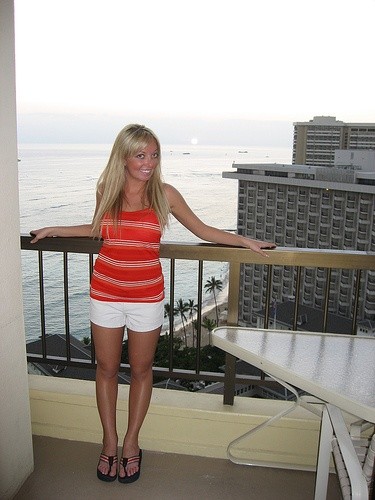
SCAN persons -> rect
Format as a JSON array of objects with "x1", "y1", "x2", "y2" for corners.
[{"x1": 30, "y1": 124, "x2": 276, "y2": 483}]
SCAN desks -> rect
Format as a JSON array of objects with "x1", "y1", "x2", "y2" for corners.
[{"x1": 211, "y1": 326, "x2": 375, "y2": 500}]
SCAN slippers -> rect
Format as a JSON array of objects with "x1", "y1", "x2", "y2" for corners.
[
  {"x1": 96, "y1": 454, "x2": 118, "y2": 482},
  {"x1": 118, "y1": 449, "x2": 142, "y2": 484}
]
